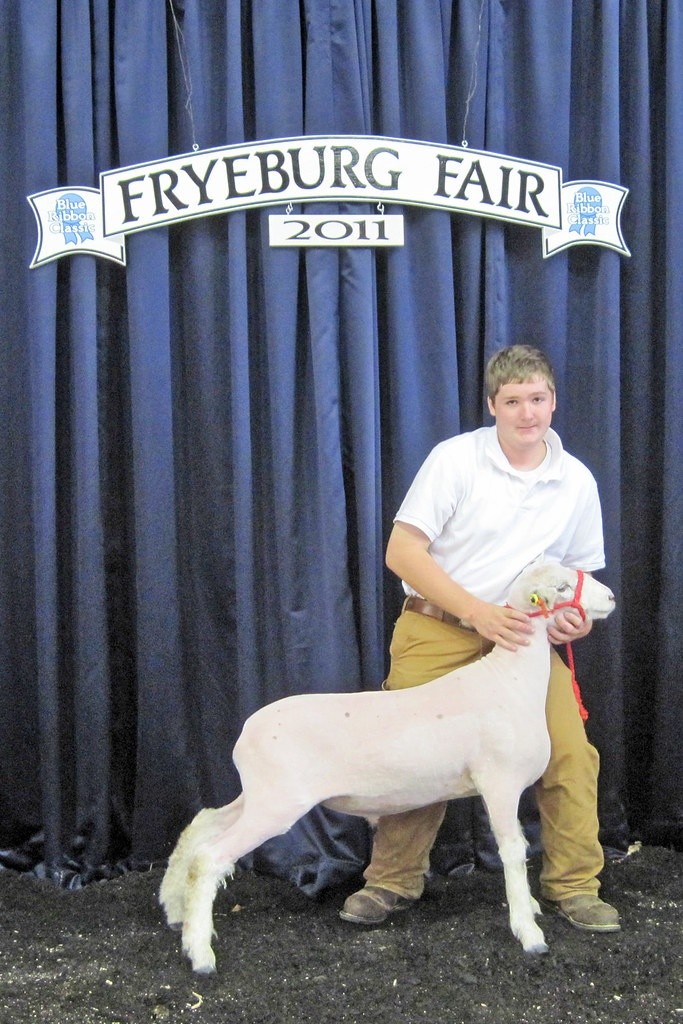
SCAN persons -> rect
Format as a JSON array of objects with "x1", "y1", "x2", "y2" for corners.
[{"x1": 338, "y1": 344, "x2": 624, "y2": 933}]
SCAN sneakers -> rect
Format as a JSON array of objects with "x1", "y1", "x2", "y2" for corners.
[
  {"x1": 539, "y1": 894, "x2": 621, "y2": 933},
  {"x1": 338, "y1": 886, "x2": 414, "y2": 924}
]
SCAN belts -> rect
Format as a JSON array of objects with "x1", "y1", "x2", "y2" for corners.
[{"x1": 405, "y1": 597, "x2": 478, "y2": 633}]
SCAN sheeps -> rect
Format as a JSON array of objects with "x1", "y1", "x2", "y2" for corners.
[{"x1": 160, "y1": 560, "x2": 617, "y2": 978}]
